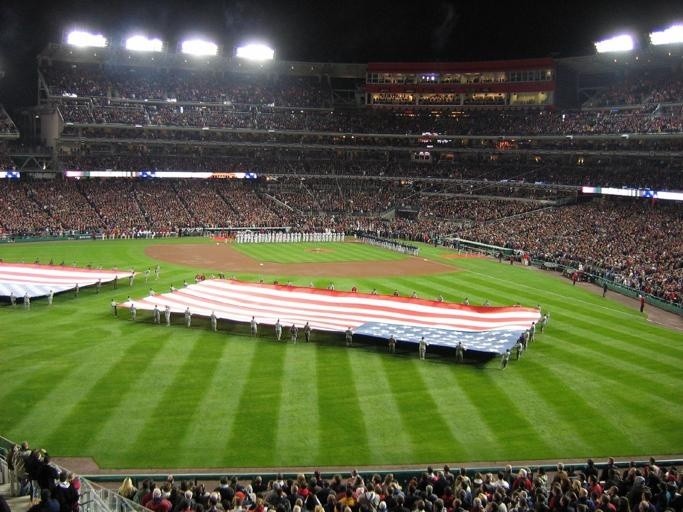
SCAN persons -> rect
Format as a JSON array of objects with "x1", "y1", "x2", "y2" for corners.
[
  {"x1": 0, "y1": 439, "x2": 80, "y2": 510},
  {"x1": 0, "y1": 256, "x2": 161, "y2": 311}
]
